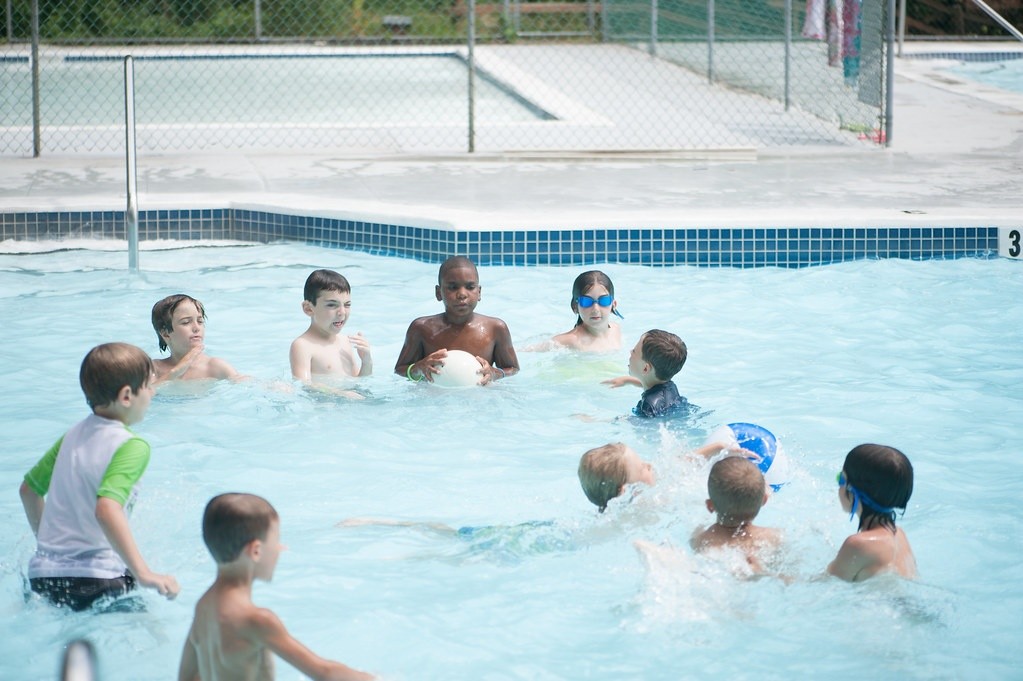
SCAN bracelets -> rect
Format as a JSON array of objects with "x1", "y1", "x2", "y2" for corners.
[
  {"x1": 406, "y1": 365, "x2": 421, "y2": 382},
  {"x1": 497, "y1": 368, "x2": 506, "y2": 378}
]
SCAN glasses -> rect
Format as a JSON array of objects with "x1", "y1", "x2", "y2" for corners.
[
  {"x1": 837, "y1": 470, "x2": 848, "y2": 487},
  {"x1": 575, "y1": 295, "x2": 612, "y2": 308}
]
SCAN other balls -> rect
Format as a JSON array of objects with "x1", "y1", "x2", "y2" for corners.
[{"x1": 427, "y1": 349, "x2": 484, "y2": 390}]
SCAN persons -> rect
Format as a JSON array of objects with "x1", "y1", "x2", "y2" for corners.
[
  {"x1": 176, "y1": 493, "x2": 379, "y2": 681},
  {"x1": 393, "y1": 256, "x2": 519, "y2": 385},
  {"x1": 556, "y1": 270, "x2": 625, "y2": 351},
  {"x1": 576, "y1": 330, "x2": 800, "y2": 574},
  {"x1": 291, "y1": 268, "x2": 374, "y2": 380},
  {"x1": 19, "y1": 343, "x2": 181, "y2": 617},
  {"x1": 826, "y1": 445, "x2": 917, "y2": 583},
  {"x1": 142, "y1": 293, "x2": 250, "y2": 380}
]
